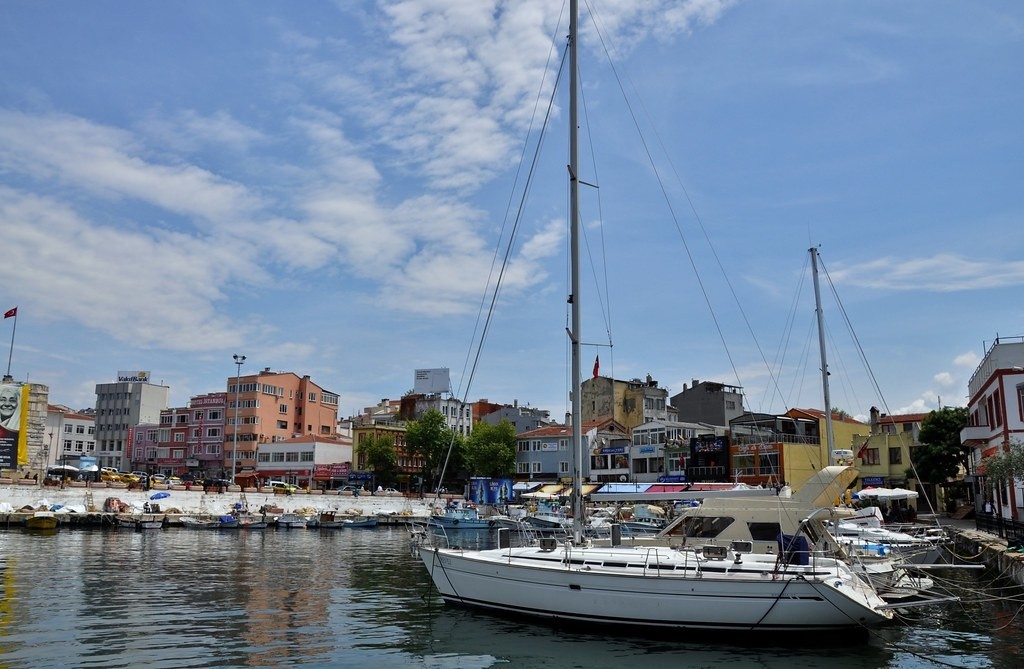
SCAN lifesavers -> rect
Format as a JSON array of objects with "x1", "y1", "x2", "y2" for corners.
[
  {"x1": 489, "y1": 520, "x2": 496, "y2": 527},
  {"x1": 453, "y1": 519, "x2": 460, "y2": 524}
]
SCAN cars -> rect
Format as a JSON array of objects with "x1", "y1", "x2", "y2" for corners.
[
  {"x1": 336, "y1": 486, "x2": 361, "y2": 497},
  {"x1": 376, "y1": 488, "x2": 398, "y2": 496},
  {"x1": 100, "y1": 466, "x2": 304, "y2": 495},
  {"x1": 47, "y1": 467, "x2": 84, "y2": 481}
]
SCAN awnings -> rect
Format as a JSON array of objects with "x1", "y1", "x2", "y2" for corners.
[
  {"x1": 973, "y1": 459, "x2": 989, "y2": 478},
  {"x1": 234, "y1": 473, "x2": 260, "y2": 478},
  {"x1": 511, "y1": 482, "x2": 738, "y2": 497}
]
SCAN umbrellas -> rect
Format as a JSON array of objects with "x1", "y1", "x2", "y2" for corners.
[
  {"x1": 840, "y1": 485, "x2": 919, "y2": 511},
  {"x1": 521, "y1": 491, "x2": 559, "y2": 505},
  {"x1": 41, "y1": 464, "x2": 107, "y2": 474}
]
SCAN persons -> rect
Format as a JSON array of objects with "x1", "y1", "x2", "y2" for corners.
[
  {"x1": 0, "y1": 384, "x2": 22, "y2": 429},
  {"x1": 434, "y1": 485, "x2": 450, "y2": 494},
  {"x1": 245, "y1": 477, "x2": 262, "y2": 493},
  {"x1": 881, "y1": 504, "x2": 916, "y2": 523},
  {"x1": 138, "y1": 475, "x2": 194, "y2": 491},
  {"x1": 0, "y1": 467, "x2": 90, "y2": 487},
  {"x1": 982, "y1": 497, "x2": 997, "y2": 534},
  {"x1": 360, "y1": 483, "x2": 386, "y2": 493}
]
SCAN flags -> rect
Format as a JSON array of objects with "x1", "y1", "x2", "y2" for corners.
[{"x1": 592, "y1": 354, "x2": 600, "y2": 379}]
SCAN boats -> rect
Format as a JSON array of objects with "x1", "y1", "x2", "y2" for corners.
[
  {"x1": 115, "y1": 516, "x2": 162, "y2": 530},
  {"x1": 23, "y1": 517, "x2": 58, "y2": 530},
  {"x1": 178, "y1": 513, "x2": 377, "y2": 529}
]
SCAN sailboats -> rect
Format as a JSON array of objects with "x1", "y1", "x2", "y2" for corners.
[{"x1": 409, "y1": 0, "x2": 963, "y2": 631}]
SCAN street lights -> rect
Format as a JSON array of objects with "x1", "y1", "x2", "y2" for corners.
[{"x1": 233, "y1": 354, "x2": 247, "y2": 485}]
